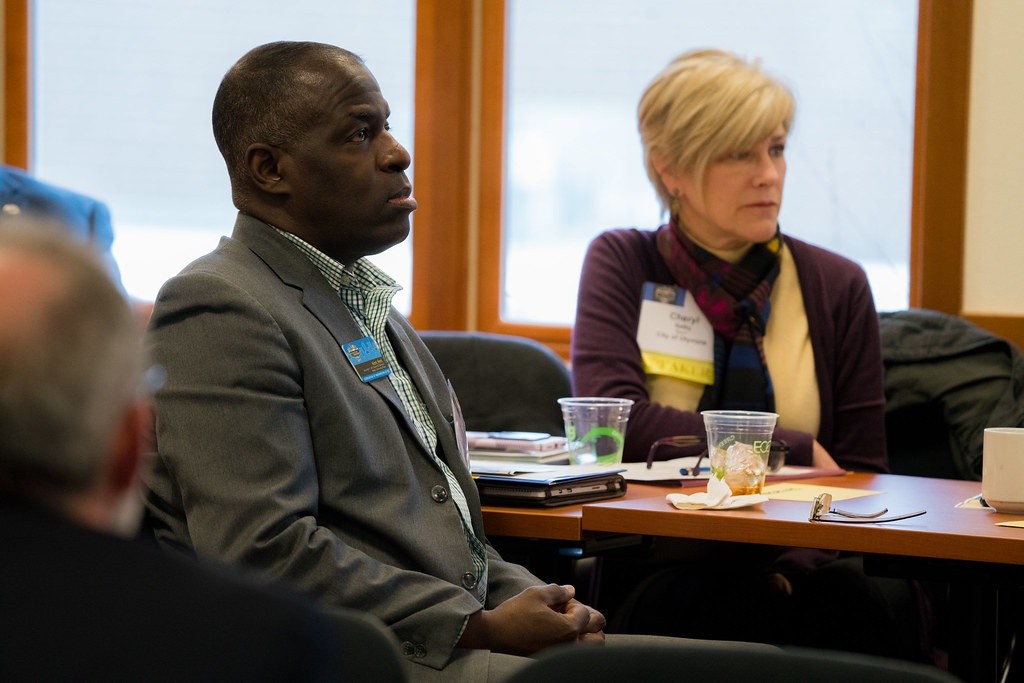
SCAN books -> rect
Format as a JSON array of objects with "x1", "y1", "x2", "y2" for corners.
[{"x1": 467, "y1": 430, "x2": 628, "y2": 510}]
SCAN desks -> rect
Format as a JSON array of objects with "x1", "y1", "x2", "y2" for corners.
[{"x1": 467, "y1": 460, "x2": 1024, "y2": 683}]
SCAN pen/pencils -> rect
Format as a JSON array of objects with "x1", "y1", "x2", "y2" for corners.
[{"x1": 679, "y1": 467, "x2": 711, "y2": 476}]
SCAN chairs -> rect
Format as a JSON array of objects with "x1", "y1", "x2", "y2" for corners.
[{"x1": 413, "y1": 330, "x2": 574, "y2": 441}]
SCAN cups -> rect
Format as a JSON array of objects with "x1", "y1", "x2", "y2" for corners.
[
  {"x1": 701, "y1": 409, "x2": 780, "y2": 496},
  {"x1": 559, "y1": 396, "x2": 634, "y2": 467},
  {"x1": 981, "y1": 427, "x2": 1024, "y2": 514}
]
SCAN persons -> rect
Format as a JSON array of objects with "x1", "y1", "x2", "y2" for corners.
[
  {"x1": 132, "y1": 44, "x2": 782, "y2": 683},
  {"x1": 0, "y1": 214, "x2": 409, "y2": 683},
  {"x1": 571, "y1": 48, "x2": 952, "y2": 672}
]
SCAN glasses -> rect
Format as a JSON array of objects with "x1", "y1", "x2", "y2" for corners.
[
  {"x1": 645, "y1": 436, "x2": 791, "y2": 476},
  {"x1": 807, "y1": 491, "x2": 928, "y2": 527}
]
